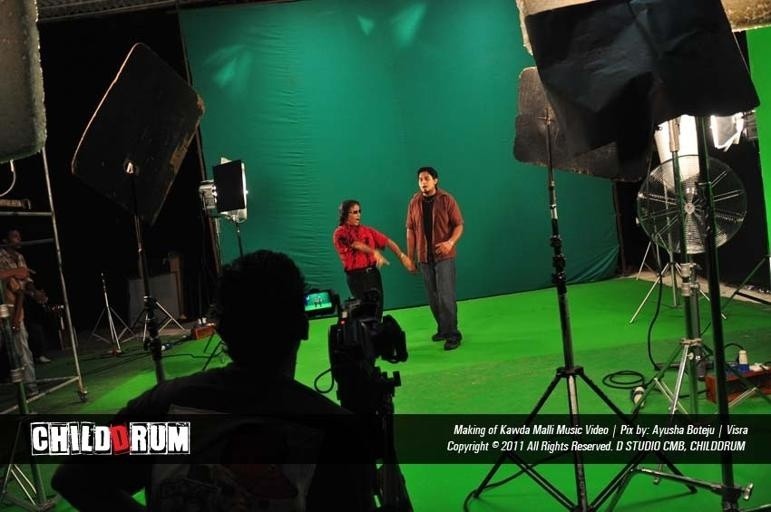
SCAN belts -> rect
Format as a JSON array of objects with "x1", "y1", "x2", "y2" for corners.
[{"x1": 347, "y1": 266, "x2": 376, "y2": 275}]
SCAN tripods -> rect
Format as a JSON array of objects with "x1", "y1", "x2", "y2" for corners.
[
  {"x1": 627, "y1": 261, "x2": 771, "y2": 483},
  {"x1": 630, "y1": 188, "x2": 727, "y2": 324},
  {"x1": 90, "y1": 271, "x2": 135, "y2": 354},
  {"x1": 127, "y1": 228, "x2": 187, "y2": 347},
  {"x1": 475, "y1": 237, "x2": 695, "y2": 512}
]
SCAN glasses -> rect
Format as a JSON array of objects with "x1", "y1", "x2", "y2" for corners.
[{"x1": 344, "y1": 209, "x2": 362, "y2": 215}]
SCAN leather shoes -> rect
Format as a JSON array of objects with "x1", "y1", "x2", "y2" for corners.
[
  {"x1": 432, "y1": 333, "x2": 446, "y2": 341},
  {"x1": 444, "y1": 340, "x2": 458, "y2": 350}
]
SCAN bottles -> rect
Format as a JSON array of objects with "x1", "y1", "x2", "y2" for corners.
[
  {"x1": 633, "y1": 387, "x2": 647, "y2": 408},
  {"x1": 738, "y1": 350, "x2": 749, "y2": 371},
  {"x1": 161, "y1": 343, "x2": 172, "y2": 352}
]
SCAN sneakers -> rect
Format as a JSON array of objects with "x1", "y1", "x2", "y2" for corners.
[{"x1": 34, "y1": 355, "x2": 52, "y2": 363}]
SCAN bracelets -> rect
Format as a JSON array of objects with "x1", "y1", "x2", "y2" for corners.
[
  {"x1": 397, "y1": 253, "x2": 405, "y2": 261},
  {"x1": 447, "y1": 240, "x2": 456, "y2": 247}
]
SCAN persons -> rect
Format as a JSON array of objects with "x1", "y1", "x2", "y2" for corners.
[
  {"x1": 1, "y1": 277, "x2": 39, "y2": 397},
  {"x1": 51, "y1": 248, "x2": 376, "y2": 512},
  {"x1": 407, "y1": 167, "x2": 464, "y2": 350},
  {"x1": 0, "y1": 229, "x2": 51, "y2": 363},
  {"x1": 333, "y1": 199, "x2": 417, "y2": 318}
]
